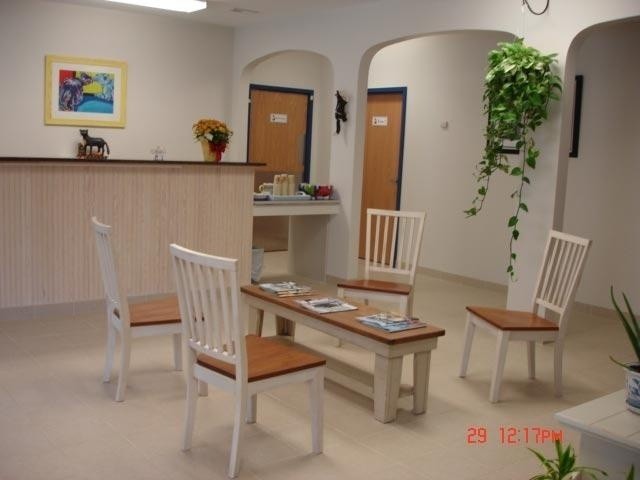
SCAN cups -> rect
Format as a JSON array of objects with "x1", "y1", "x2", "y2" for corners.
[{"x1": 258, "y1": 174, "x2": 332, "y2": 200}]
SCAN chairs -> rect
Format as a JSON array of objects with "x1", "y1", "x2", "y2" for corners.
[
  {"x1": 335, "y1": 205, "x2": 425, "y2": 351},
  {"x1": 168, "y1": 241, "x2": 326, "y2": 479},
  {"x1": 460, "y1": 226, "x2": 593, "y2": 406},
  {"x1": 89, "y1": 214, "x2": 210, "y2": 403}
]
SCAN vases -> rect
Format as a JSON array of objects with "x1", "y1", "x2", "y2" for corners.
[{"x1": 200, "y1": 141, "x2": 222, "y2": 163}]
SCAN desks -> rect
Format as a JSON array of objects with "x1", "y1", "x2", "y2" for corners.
[{"x1": 240, "y1": 282, "x2": 445, "y2": 425}]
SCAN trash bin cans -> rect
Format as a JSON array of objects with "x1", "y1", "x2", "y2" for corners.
[{"x1": 252, "y1": 247, "x2": 266, "y2": 281}]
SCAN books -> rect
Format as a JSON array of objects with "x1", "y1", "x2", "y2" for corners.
[
  {"x1": 260, "y1": 281, "x2": 319, "y2": 298},
  {"x1": 356, "y1": 310, "x2": 428, "y2": 333},
  {"x1": 293, "y1": 298, "x2": 358, "y2": 314}
]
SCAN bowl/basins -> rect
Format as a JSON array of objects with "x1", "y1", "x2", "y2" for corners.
[{"x1": 253, "y1": 194, "x2": 268, "y2": 200}]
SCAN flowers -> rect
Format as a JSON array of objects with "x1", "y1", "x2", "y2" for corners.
[{"x1": 191, "y1": 118, "x2": 234, "y2": 162}]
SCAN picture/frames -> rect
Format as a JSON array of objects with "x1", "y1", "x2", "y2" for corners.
[{"x1": 42, "y1": 53, "x2": 131, "y2": 129}]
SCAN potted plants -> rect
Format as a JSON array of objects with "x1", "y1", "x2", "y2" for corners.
[{"x1": 603, "y1": 284, "x2": 639, "y2": 416}]
